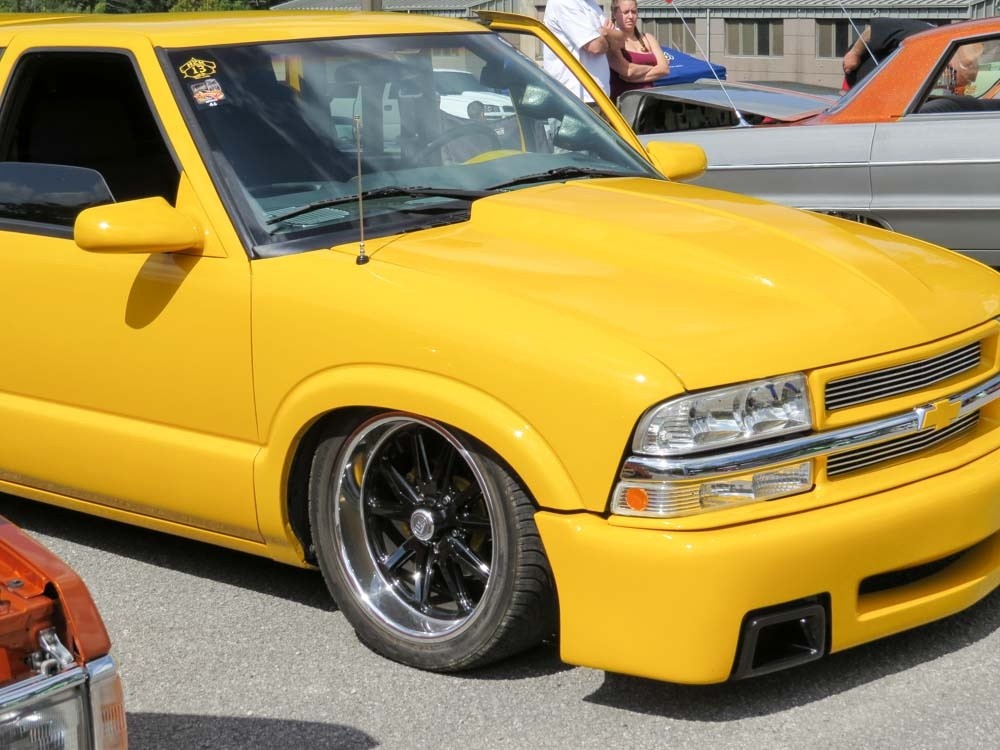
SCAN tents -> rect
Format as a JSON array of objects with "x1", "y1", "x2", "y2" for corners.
[{"x1": 652, "y1": 46, "x2": 726, "y2": 87}]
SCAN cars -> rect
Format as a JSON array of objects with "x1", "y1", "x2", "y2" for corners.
[
  {"x1": 0, "y1": 504, "x2": 129, "y2": 750},
  {"x1": 2, "y1": 11, "x2": 1000, "y2": 686}
]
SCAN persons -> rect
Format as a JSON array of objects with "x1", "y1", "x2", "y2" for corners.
[
  {"x1": 608, "y1": 0, "x2": 670, "y2": 106},
  {"x1": 842, "y1": 16, "x2": 985, "y2": 95},
  {"x1": 543, "y1": 0, "x2": 626, "y2": 157},
  {"x1": 467, "y1": 101, "x2": 486, "y2": 121}
]
